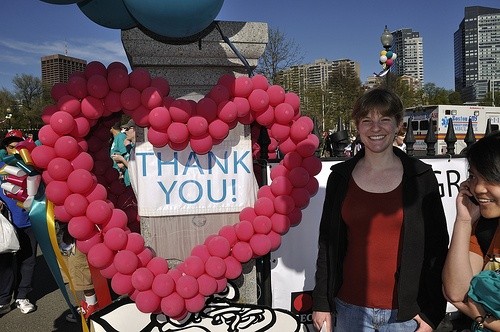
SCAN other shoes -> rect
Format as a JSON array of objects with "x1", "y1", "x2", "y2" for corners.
[{"x1": 66, "y1": 301, "x2": 98, "y2": 322}]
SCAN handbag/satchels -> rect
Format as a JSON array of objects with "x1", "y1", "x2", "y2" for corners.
[
  {"x1": 453, "y1": 270, "x2": 500, "y2": 332},
  {"x1": 0, "y1": 213, "x2": 21, "y2": 254}
]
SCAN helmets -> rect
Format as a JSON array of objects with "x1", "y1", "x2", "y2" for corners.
[{"x1": 3, "y1": 130, "x2": 25, "y2": 146}]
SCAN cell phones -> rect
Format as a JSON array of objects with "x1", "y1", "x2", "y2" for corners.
[{"x1": 467, "y1": 187, "x2": 480, "y2": 206}]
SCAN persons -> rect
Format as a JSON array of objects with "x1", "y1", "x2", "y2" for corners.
[
  {"x1": 312, "y1": 90, "x2": 449, "y2": 331},
  {"x1": 0, "y1": 117, "x2": 138, "y2": 315},
  {"x1": 443, "y1": 132, "x2": 500, "y2": 332}
]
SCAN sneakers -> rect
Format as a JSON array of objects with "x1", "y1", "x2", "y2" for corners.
[
  {"x1": 15, "y1": 298, "x2": 35, "y2": 314},
  {"x1": 0, "y1": 304, "x2": 11, "y2": 315}
]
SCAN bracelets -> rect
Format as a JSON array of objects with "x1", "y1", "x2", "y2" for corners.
[{"x1": 474, "y1": 309, "x2": 489, "y2": 331}]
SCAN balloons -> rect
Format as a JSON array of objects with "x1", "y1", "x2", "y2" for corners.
[
  {"x1": 379, "y1": 48, "x2": 399, "y2": 67},
  {"x1": 77, "y1": 0, "x2": 225, "y2": 40},
  {"x1": 33, "y1": 61, "x2": 322, "y2": 320}
]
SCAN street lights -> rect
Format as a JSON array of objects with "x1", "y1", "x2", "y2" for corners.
[
  {"x1": 380, "y1": 24, "x2": 394, "y2": 89},
  {"x1": 6, "y1": 106, "x2": 12, "y2": 130}
]
